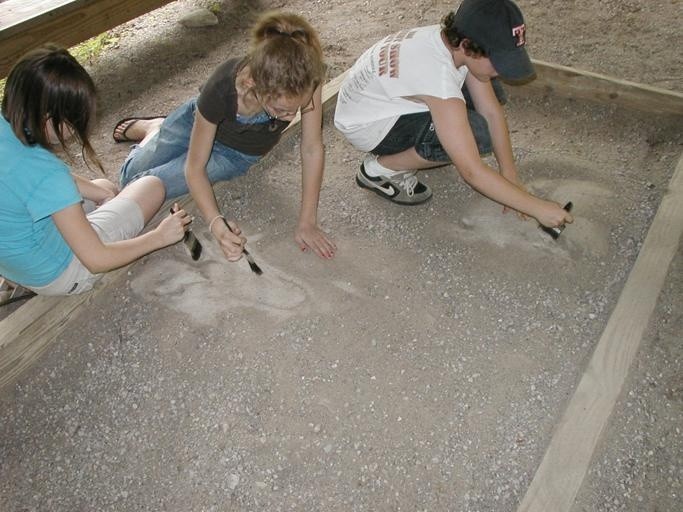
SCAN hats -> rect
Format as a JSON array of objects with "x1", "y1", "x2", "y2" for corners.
[{"x1": 449, "y1": 0, "x2": 538, "y2": 87}]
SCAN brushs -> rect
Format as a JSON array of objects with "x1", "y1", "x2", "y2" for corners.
[
  {"x1": 222, "y1": 218, "x2": 263, "y2": 276},
  {"x1": 537, "y1": 202, "x2": 572, "y2": 240},
  {"x1": 170, "y1": 208, "x2": 202, "y2": 261}
]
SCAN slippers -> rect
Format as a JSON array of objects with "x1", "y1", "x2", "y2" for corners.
[{"x1": 113, "y1": 116, "x2": 167, "y2": 142}]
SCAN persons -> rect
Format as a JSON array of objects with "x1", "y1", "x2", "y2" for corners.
[
  {"x1": 111, "y1": 10, "x2": 340, "y2": 265},
  {"x1": 331, "y1": 0, "x2": 574, "y2": 230},
  {"x1": 0, "y1": 42, "x2": 196, "y2": 297}
]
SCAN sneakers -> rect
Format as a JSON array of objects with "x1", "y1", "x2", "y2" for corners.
[
  {"x1": 356, "y1": 155, "x2": 434, "y2": 206},
  {"x1": 0, "y1": 280, "x2": 34, "y2": 307}
]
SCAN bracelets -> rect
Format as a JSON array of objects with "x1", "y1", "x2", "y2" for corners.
[{"x1": 207, "y1": 214, "x2": 224, "y2": 233}]
